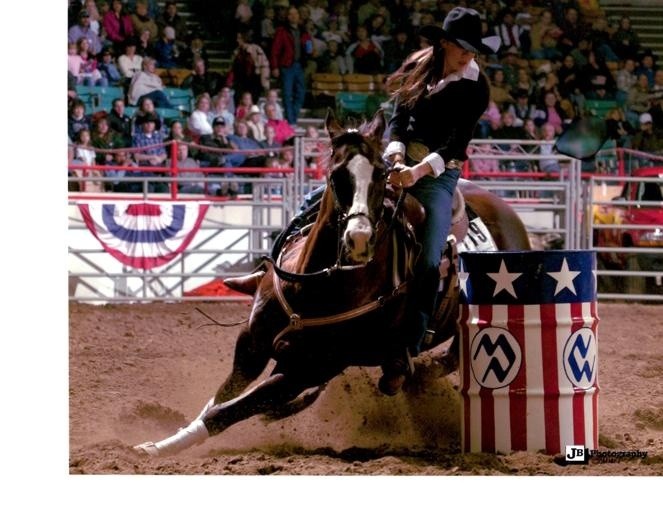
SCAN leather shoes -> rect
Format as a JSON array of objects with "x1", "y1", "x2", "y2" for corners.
[
  {"x1": 378, "y1": 364, "x2": 409, "y2": 396},
  {"x1": 223, "y1": 271, "x2": 266, "y2": 295}
]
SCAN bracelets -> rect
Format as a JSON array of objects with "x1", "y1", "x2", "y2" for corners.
[{"x1": 391, "y1": 159, "x2": 405, "y2": 166}]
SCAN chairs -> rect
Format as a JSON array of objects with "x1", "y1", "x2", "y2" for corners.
[{"x1": 68, "y1": 62, "x2": 663, "y2": 195}]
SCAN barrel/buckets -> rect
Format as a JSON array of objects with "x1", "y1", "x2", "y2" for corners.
[{"x1": 456, "y1": 249, "x2": 600, "y2": 458}]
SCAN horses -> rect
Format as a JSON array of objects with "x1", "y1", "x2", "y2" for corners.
[{"x1": 132, "y1": 106, "x2": 534, "y2": 460}]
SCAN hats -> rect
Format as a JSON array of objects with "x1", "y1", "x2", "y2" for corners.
[
  {"x1": 248, "y1": 105, "x2": 261, "y2": 116},
  {"x1": 79, "y1": 10, "x2": 89, "y2": 17},
  {"x1": 418, "y1": 7, "x2": 502, "y2": 55},
  {"x1": 163, "y1": 26, "x2": 175, "y2": 39},
  {"x1": 213, "y1": 116, "x2": 225, "y2": 124},
  {"x1": 640, "y1": 113, "x2": 653, "y2": 124},
  {"x1": 137, "y1": 114, "x2": 161, "y2": 130}
]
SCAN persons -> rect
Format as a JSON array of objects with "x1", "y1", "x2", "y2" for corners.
[
  {"x1": 223, "y1": 8, "x2": 489, "y2": 396},
  {"x1": 67, "y1": 3, "x2": 661, "y2": 202}
]
